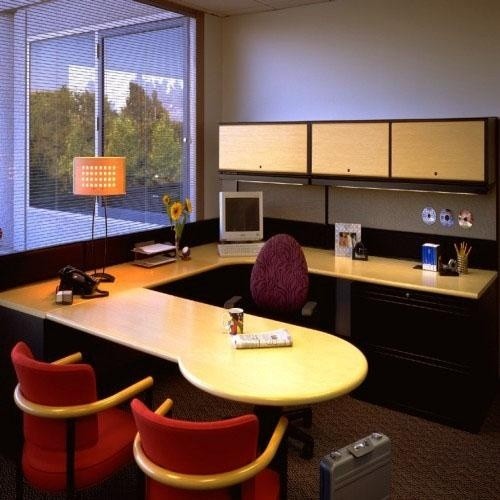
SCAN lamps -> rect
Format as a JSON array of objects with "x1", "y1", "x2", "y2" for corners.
[{"x1": 73, "y1": 156, "x2": 126, "y2": 283}]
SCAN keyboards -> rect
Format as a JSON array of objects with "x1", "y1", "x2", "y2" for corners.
[{"x1": 217, "y1": 243, "x2": 265, "y2": 257}]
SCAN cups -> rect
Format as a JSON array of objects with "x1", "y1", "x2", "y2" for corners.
[{"x1": 228, "y1": 308, "x2": 243, "y2": 335}]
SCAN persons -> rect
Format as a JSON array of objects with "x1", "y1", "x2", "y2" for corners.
[{"x1": 339, "y1": 232, "x2": 356, "y2": 248}]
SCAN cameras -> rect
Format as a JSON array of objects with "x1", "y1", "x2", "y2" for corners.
[{"x1": 438, "y1": 256, "x2": 459, "y2": 276}]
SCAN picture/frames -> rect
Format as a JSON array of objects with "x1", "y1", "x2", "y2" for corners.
[{"x1": 335, "y1": 223, "x2": 362, "y2": 258}]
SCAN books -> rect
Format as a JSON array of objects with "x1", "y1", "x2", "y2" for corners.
[
  {"x1": 421, "y1": 242, "x2": 441, "y2": 272},
  {"x1": 232, "y1": 327, "x2": 294, "y2": 349}
]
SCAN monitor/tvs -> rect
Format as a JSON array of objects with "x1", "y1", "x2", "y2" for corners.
[{"x1": 219, "y1": 191, "x2": 264, "y2": 242}]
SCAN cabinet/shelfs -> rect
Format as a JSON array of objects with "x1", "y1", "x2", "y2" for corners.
[
  {"x1": 391, "y1": 118, "x2": 499, "y2": 196},
  {"x1": 217, "y1": 122, "x2": 310, "y2": 188},
  {"x1": 310, "y1": 120, "x2": 390, "y2": 191}
]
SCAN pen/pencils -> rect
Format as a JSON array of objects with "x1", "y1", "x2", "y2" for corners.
[{"x1": 454, "y1": 241, "x2": 472, "y2": 256}]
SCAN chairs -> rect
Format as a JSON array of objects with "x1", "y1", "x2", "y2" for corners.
[
  {"x1": 223, "y1": 232, "x2": 322, "y2": 461},
  {"x1": 128, "y1": 398, "x2": 290, "y2": 500},
  {"x1": 11, "y1": 341, "x2": 155, "y2": 499}
]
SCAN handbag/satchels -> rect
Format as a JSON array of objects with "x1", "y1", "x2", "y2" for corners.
[{"x1": 319, "y1": 433, "x2": 391, "y2": 500}]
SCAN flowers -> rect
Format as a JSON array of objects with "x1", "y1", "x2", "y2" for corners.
[{"x1": 162, "y1": 194, "x2": 193, "y2": 239}]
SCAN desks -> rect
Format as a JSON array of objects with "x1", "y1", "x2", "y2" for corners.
[
  {"x1": 46, "y1": 287, "x2": 370, "y2": 499},
  {"x1": 0, "y1": 239, "x2": 498, "y2": 430}
]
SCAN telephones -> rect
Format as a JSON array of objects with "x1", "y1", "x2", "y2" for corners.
[{"x1": 56, "y1": 265, "x2": 100, "y2": 295}]
